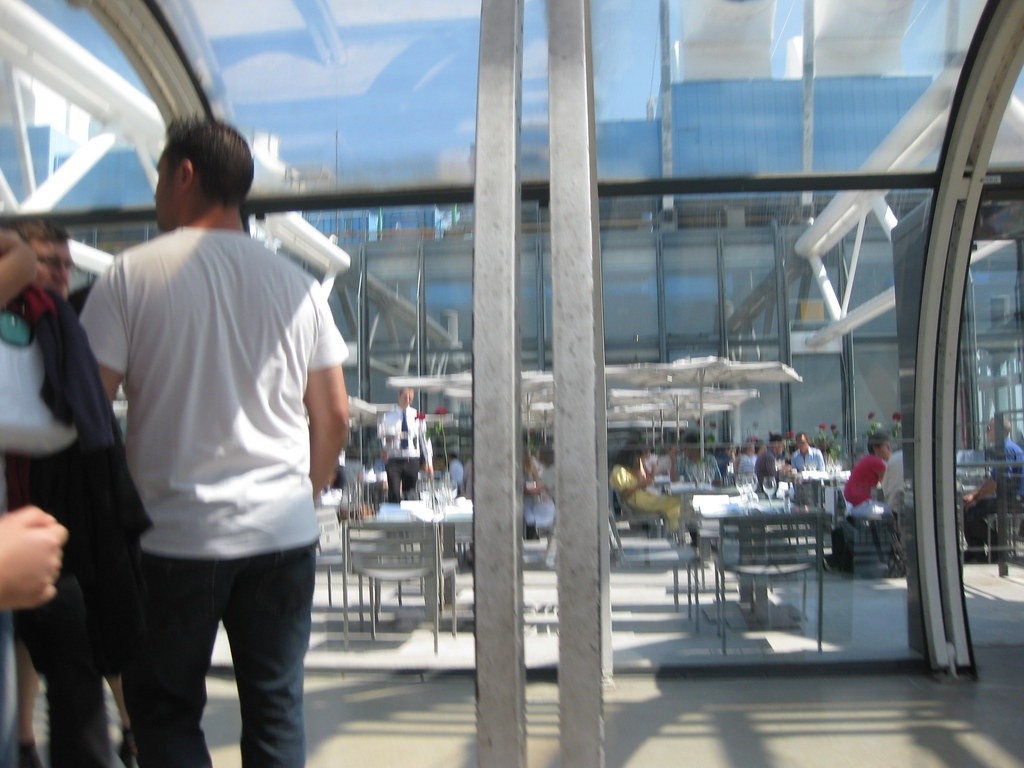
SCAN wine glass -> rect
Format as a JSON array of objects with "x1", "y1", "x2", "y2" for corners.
[
  {"x1": 417, "y1": 469, "x2": 457, "y2": 520},
  {"x1": 342, "y1": 463, "x2": 388, "y2": 524},
  {"x1": 683, "y1": 461, "x2": 781, "y2": 515},
  {"x1": 801, "y1": 459, "x2": 843, "y2": 477}
]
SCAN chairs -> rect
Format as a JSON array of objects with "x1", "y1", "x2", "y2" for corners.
[{"x1": 312, "y1": 450, "x2": 1024, "y2": 654}]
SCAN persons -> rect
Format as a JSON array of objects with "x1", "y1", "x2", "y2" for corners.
[
  {"x1": 883, "y1": 448, "x2": 904, "y2": 512},
  {"x1": 963, "y1": 415, "x2": 1023, "y2": 562},
  {"x1": 349, "y1": 426, "x2": 767, "y2": 543},
  {"x1": 790, "y1": 433, "x2": 826, "y2": 472},
  {"x1": 76, "y1": 119, "x2": 347, "y2": 766},
  {"x1": 845, "y1": 432, "x2": 897, "y2": 528},
  {"x1": 19, "y1": 220, "x2": 73, "y2": 302},
  {"x1": 0, "y1": 226, "x2": 139, "y2": 767},
  {"x1": 755, "y1": 433, "x2": 791, "y2": 497},
  {"x1": 377, "y1": 387, "x2": 434, "y2": 503}
]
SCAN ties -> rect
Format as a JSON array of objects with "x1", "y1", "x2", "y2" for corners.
[{"x1": 400, "y1": 410, "x2": 408, "y2": 450}]
